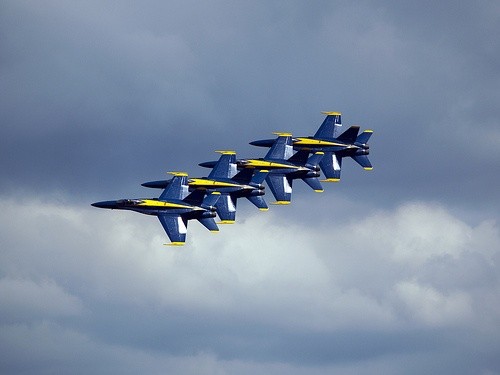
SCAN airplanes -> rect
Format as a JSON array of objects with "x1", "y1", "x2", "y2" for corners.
[{"x1": 90, "y1": 111, "x2": 373, "y2": 245}]
